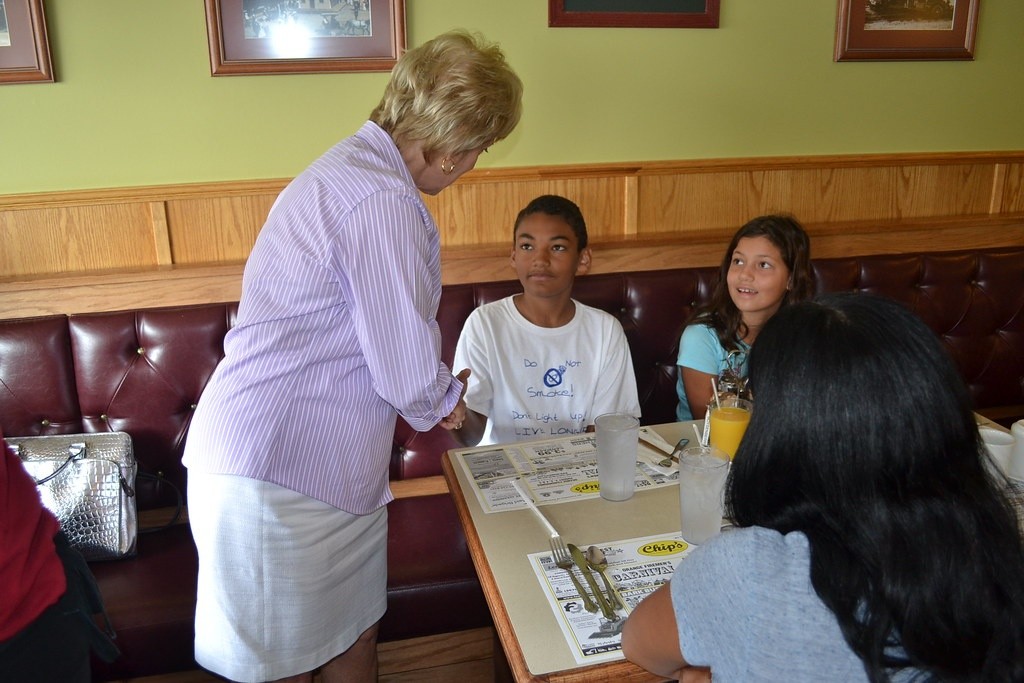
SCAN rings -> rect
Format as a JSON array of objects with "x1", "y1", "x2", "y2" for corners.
[{"x1": 454, "y1": 422, "x2": 462, "y2": 430}]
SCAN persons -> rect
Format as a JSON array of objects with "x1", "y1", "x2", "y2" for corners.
[
  {"x1": 675, "y1": 215, "x2": 812, "y2": 423},
  {"x1": 180, "y1": 29, "x2": 524, "y2": 683},
  {"x1": 452, "y1": 195, "x2": 642, "y2": 447},
  {"x1": 622, "y1": 291, "x2": 1024, "y2": 683},
  {"x1": 0, "y1": 433, "x2": 122, "y2": 683}
]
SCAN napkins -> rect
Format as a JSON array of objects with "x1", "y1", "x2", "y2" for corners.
[{"x1": 635, "y1": 431, "x2": 681, "y2": 477}]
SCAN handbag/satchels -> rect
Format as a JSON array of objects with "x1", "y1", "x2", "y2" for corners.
[{"x1": 2, "y1": 431, "x2": 139, "y2": 561}]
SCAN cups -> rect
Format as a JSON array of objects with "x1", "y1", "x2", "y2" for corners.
[
  {"x1": 977, "y1": 429, "x2": 1016, "y2": 492},
  {"x1": 708, "y1": 398, "x2": 754, "y2": 462},
  {"x1": 1007, "y1": 420, "x2": 1024, "y2": 483},
  {"x1": 594, "y1": 412, "x2": 639, "y2": 502},
  {"x1": 678, "y1": 446, "x2": 730, "y2": 546}
]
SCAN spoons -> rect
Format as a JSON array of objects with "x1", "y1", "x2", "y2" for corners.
[{"x1": 587, "y1": 546, "x2": 632, "y2": 616}]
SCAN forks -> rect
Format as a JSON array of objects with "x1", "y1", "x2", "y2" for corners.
[
  {"x1": 657, "y1": 439, "x2": 689, "y2": 467},
  {"x1": 549, "y1": 538, "x2": 599, "y2": 613}
]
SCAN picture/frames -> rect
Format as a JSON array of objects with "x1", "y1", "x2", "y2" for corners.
[
  {"x1": 833, "y1": 0, "x2": 981, "y2": 62},
  {"x1": 202, "y1": 0, "x2": 407, "y2": 77},
  {"x1": 0, "y1": 0, "x2": 56, "y2": 85},
  {"x1": 548, "y1": 0, "x2": 720, "y2": 28}
]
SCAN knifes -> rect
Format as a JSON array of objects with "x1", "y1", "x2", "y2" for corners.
[{"x1": 567, "y1": 543, "x2": 616, "y2": 622}]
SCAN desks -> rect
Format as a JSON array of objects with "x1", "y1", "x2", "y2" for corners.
[{"x1": 440, "y1": 412, "x2": 1024, "y2": 683}]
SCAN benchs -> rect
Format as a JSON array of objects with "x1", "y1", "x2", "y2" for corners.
[{"x1": 0, "y1": 245, "x2": 1024, "y2": 683}]
